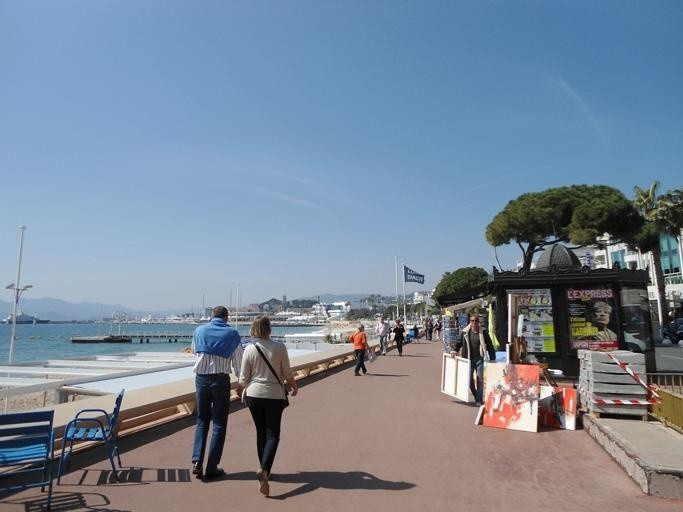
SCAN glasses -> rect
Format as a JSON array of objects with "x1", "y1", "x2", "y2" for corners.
[{"x1": 470, "y1": 320, "x2": 477, "y2": 322}]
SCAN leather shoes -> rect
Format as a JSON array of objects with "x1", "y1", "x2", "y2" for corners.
[
  {"x1": 257, "y1": 469, "x2": 271, "y2": 495},
  {"x1": 191, "y1": 462, "x2": 224, "y2": 481}
]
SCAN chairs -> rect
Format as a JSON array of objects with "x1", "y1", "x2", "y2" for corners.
[
  {"x1": 1, "y1": 410, "x2": 56, "y2": 511},
  {"x1": 57, "y1": 388, "x2": 126, "y2": 485},
  {"x1": 402, "y1": 328, "x2": 417, "y2": 343}
]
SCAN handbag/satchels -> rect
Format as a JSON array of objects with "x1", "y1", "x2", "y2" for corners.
[
  {"x1": 283, "y1": 389, "x2": 289, "y2": 408},
  {"x1": 368, "y1": 352, "x2": 376, "y2": 361}
]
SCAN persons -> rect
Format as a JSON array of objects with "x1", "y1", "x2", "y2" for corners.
[
  {"x1": 236, "y1": 316, "x2": 298, "y2": 495},
  {"x1": 411, "y1": 318, "x2": 442, "y2": 340},
  {"x1": 348, "y1": 323, "x2": 370, "y2": 376},
  {"x1": 374, "y1": 317, "x2": 392, "y2": 357},
  {"x1": 578, "y1": 300, "x2": 618, "y2": 341},
  {"x1": 190, "y1": 307, "x2": 246, "y2": 481},
  {"x1": 451, "y1": 314, "x2": 496, "y2": 407},
  {"x1": 392, "y1": 319, "x2": 405, "y2": 356}
]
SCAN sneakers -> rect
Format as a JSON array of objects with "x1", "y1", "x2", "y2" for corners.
[
  {"x1": 363, "y1": 370, "x2": 367, "y2": 374},
  {"x1": 354, "y1": 372, "x2": 361, "y2": 376}
]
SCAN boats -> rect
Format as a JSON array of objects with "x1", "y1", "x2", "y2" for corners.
[{"x1": 0, "y1": 310, "x2": 51, "y2": 325}]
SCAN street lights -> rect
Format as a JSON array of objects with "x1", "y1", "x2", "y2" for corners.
[{"x1": 4, "y1": 223, "x2": 34, "y2": 366}]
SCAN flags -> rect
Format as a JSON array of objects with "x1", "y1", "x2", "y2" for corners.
[{"x1": 404, "y1": 266, "x2": 425, "y2": 284}]
